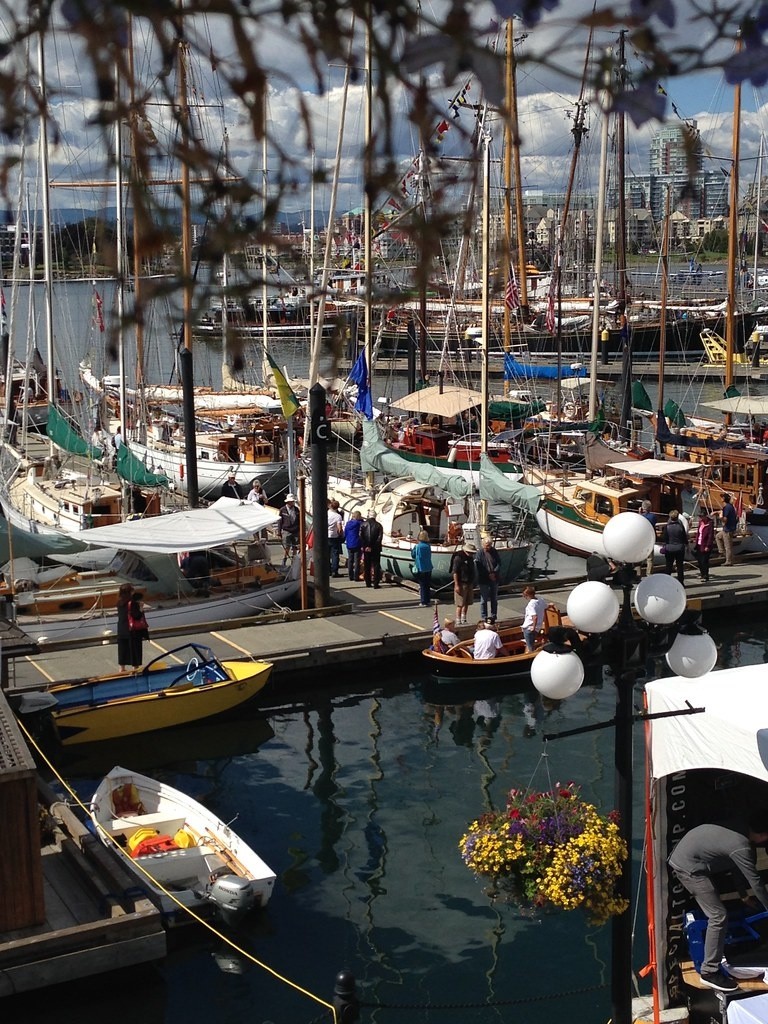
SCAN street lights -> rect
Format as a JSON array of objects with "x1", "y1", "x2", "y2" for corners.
[{"x1": 531, "y1": 500, "x2": 717, "y2": 1023}]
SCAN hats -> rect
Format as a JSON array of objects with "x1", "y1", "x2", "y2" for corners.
[
  {"x1": 720, "y1": 493, "x2": 731, "y2": 502},
  {"x1": 485, "y1": 617, "x2": 495, "y2": 625},
  {"x1": 462, "y1": 542, "x2": 477, "y2": 553},
  {"x1": 228, "y1": 473, "x2": 235, "y2": 478},
  {"x1": 419, "y1": 531, "x2": 429, "y2": 542},
  {"x1": 284, "y1": 494, "x2": 297, "y2": 502}
]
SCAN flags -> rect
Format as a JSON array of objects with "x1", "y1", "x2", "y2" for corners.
[
  {"x1": 506, "y1": 261, "x2": 521, "y2": 311},
  {"x1": 544, "y1": 290, "x2": 557, "y2": 337},
  {"x1": 264, "y1": 349, "x2": 300, "y2": 417},
  {"x1": 623, "y1": 36, "x2": 768, "y2": 233},
  {"x1": 349, "y1": 348, "x2": 373, "y2": 421},
  {"x1": 325, "y1": 16, "x2": 506, "y2": 289}
]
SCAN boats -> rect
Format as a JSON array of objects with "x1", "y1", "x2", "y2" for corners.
[
  {"x1": 90, "y1": 766, "x2": 277, "y2": 929},
  {"x1": 644, "y1": 663, "x2": 768, "y2": 1024},
  {"x1": 419, "y1": 614, "x2": 599, "y2": 681},
  {"x1": 420, "y1": 671, "x2": 540, "y2": 708},
  {"x1": 52, "y1": 710, "x2": 277, "y2": 783},
  {"x1": 48, "y1": 643, "x2": 274, "y2": 750}
]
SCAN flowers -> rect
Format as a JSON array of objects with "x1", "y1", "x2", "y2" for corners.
[{"x1": 457, "y1": 780, "x2": 628, "y2": 924}]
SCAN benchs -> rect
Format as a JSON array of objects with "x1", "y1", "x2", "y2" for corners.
[
  {"x1": 105, "y1": 812, "x2": 188, "y2": 843},
  {"x1": 135, "y1": 847, "x2": 220, "y2": 883}
]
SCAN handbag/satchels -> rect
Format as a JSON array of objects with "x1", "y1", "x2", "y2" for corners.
[
  {"x1": 660, "y1": 546, "x2": 667, "y2": 554},
  {"x1": 488, "y1": 571, "x2": 503, "y2": 582},
  {"x1": 412, "y1": 565, "x2": 418, "y2": 574},
  {"x1": 128, "y1": 601, "x2": 149, "y2": 631}
]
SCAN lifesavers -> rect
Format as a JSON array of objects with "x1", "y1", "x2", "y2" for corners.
[{"x1": 227, "y1": 414, "x2": 238, "y2": 426}]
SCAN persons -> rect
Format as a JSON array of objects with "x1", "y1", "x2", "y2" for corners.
[
  {"x1": 637, "y1": 500, "x2": 657, "y2": 577},
  {"x1": 520, "y1": 587, "x2": 549, "y2": 652},
  {"x1": 695, "y1": 510, "x2": 714, "y2": 582},
  {"x1": 247, "y1": 480, "x2": 268, "y2": 544},
  {"x1": 411, "y1": 531, "x2": 433, "y2": 607},
  {"x1": 586, "y1": 551, "x2": 611, "y2": 581},
  {"x1": 277, "y1": 494, "x2": 300, "y2": 566},
  {"x1": 91, "y1": 422, "x2": 130, "y2": 487},
  {"x1": 668, "y1": 824, "x2": 768, "y2": 992},
  {"x1": 327, "y1": 498, "x2": 383, "y2": 589},
  {"x1": 475, "y1": 536, "x2": 502, "y2": 623},
  {"x1": 473, "y1": 617, "x2": 510, "y2": 660},
  {"x1": 715, "y1": 493, "x2": 737, "y2": 566},
  {"x1": 681, "y1": 479, "x2": 704, "y2": 516},
  {"x1": 440, "y1": 617, "x2": 470, "y2": 658},
  {"x1": 448, "y1": 543, "x2": 475, "y2": 624},
  {"x1": 117, "y1": 583, "x2": 150, "y2": 673},
  {"x1": 660, "y1": 511, "x2": 688, "y2": 589},
  {"x1": 222, "y1": 464, "x2": 240, "y2": 499}
]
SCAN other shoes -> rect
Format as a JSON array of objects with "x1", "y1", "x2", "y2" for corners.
[
  {"x1": 456, "y1": 620, "x2": 468, "y2": 626},
  {"x1": 332, "y1": 574, "x2": 344, "y2": 578},
  {"x1": 349, "y1": 578, "x2": 364, "y2": 582},
  {"x1": 721, "y1": 561, "x2": 733, "y2": 566},
  {"x1": 366, "y1": 584, "x2": 381, "y2": 589},
  {"x1": 696, "y1": 575, "x2": 703, "y2": 579},
  {"x1": 701, "y1": 578, "x2": 709, "y2": 582}
]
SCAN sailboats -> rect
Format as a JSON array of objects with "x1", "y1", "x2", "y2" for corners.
[{"x1": 0, "y1": 3, "x2": 768, "y2": 654}]
